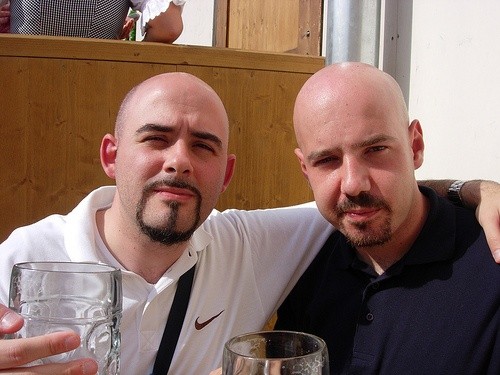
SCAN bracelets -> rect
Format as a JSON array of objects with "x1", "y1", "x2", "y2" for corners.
[{"x1": 447, "y1": 179, "x2": 467, "y2": 200}]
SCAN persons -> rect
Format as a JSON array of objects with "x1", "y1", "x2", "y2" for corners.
[
  {"x1": 211, "y1": 62, "x2": 500, "y2": 375},
  {"x1": 0, "y1": 0, "x2": 185, "y2": 44},
  {"x1": 0, "y1": 72, "x2": 500, "y2": 375}
]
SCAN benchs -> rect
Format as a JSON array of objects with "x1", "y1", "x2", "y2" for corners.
[{"x1": 0, "y1": 31, "x2": 328, "y2": 332}]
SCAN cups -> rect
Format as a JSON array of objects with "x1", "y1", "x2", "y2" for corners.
[
  {"x1": 8, "y1": 262, "x2": 123, "y2": 375},
  {"x1": 222, "y1": 330, "x2": 329, "y2": 375}
]
